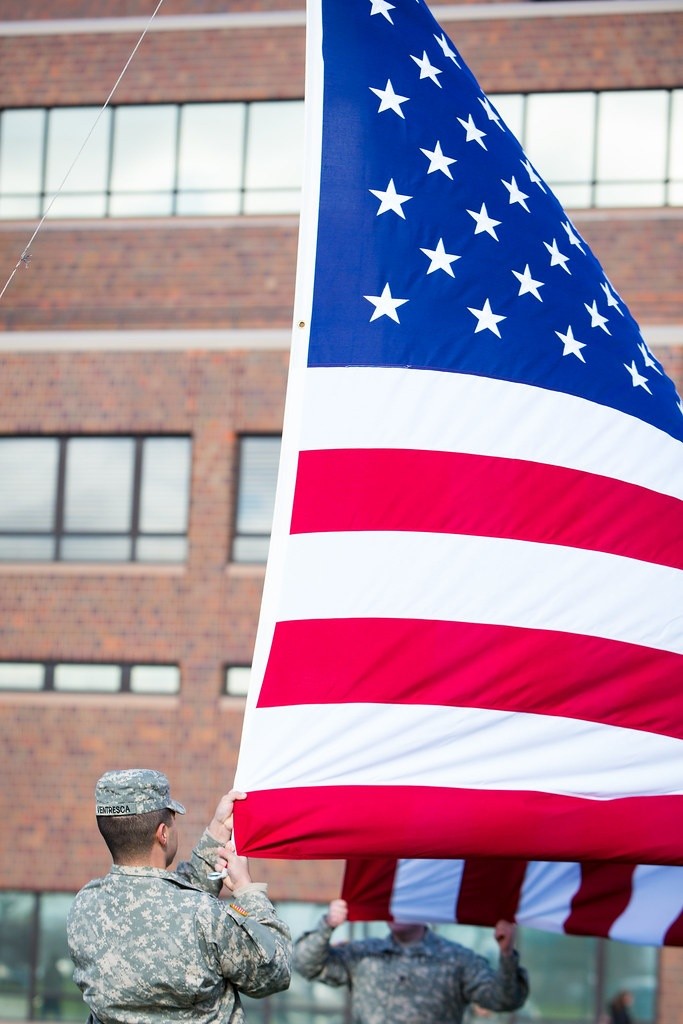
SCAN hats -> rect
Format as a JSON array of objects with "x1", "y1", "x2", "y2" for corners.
[{"x1": 94, "y1": 769, "x2": 187, "y2": 816}]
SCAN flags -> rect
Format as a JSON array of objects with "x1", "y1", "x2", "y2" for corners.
[{"x1": 232, "y1": 0, "x2": 682, "y2": 947}]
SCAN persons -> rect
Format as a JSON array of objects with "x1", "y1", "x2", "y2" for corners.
[
  {"x1": 607, "y1": 988, "x2": 639, "y2": 1024},
  {"x1": 291, "y1": 898, "x2": 529, "y2": 1024},
  {"x1": 67, "y1": 769, "x2": 292, "y2": 1024}
]
